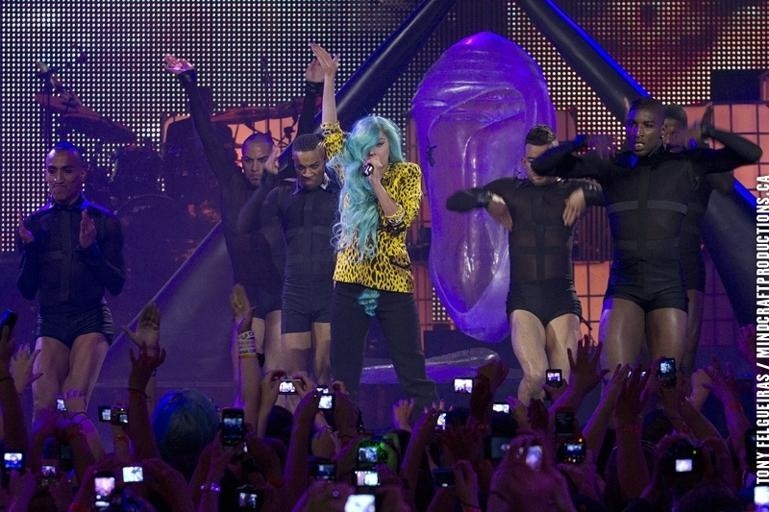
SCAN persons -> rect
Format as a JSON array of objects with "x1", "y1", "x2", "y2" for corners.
[
  {"x1": 311, "y1": 39, "x2": 438, "y2": 425},
  {"x1": 236, "y1": 135, "x2": 328, "y2": 390},
  {"x1": 2, "y1": 285, "x2": 769, "y2": 511},
  {"x1": 163, "y1": 45, "x2": 325, "y2": 414},
  {"x1": 529, "y1": 100, "x2": 762, "y2": 418},
  {"x1": 656, "y1": 100, "x2": 735, "y2": 384},
  {"x1": 14, "y1": 140, "x2": 127, "y2": 426},
  {"x1": 445, "y1": 123, "x2": 608, "y2": 412}
]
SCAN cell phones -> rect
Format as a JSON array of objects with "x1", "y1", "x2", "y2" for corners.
[
  {"x1": 276, "y1": 379, "x2": 383, "y2": 512},
  {"x1": 2, "y1": 450, "x2": 24, "y2": 490},
  {"x1": 92, "y1": 470, "x2": 116, "y2": 512},
  {"x1": 237, "y1": 484, "x2": 261, "y2": 512},
  {"x1": 122, "y1": 464, "x2": 146, "y2": 483},
  {"x1": 221, "y1": 408, "x2": 245, "y2": 446},
  {"x1": 55, "y1": 397, "x2": 67, "y2": 413},
  {"x1": 97, "y1": 406, "x2": 111, "y2": 422},
  {"x1": 39, "y1": 464, "x2": 56, "y2": 488},
  {"x1": 428, "y1": 356, "x2": 699, "y2": 491},
  {"x1": 112, "y1": 409, "x2": 128, "y2": 424}
]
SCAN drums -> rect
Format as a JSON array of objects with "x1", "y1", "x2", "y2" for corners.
[
  {"x1": 102, "y1": 179, "x2": 176, "y2": 249},
  {"x1": 109, "y1": 145, "x2": 165, "y2": 196}
]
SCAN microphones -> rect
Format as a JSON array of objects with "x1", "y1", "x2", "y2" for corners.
[{"x1": 361, "y1": 162, "x2": 374, "y2": 177}]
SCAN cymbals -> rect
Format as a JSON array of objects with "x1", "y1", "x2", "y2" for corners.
[
  {"x1": 58, "y1": 111, "x2": 137, "y2": 144},
  {"x1": 34, "y1": 90, "x2": 102, "y2": 116},
  {"x1": 210, "y1": 106, "x2": 277, "y2": 125},
  {"x1": 36, "y1": 60, "x2": 65, "y2": 91},
  {"x1": 272, "y1": 95, "x2": 322, "y2": 119}
]
